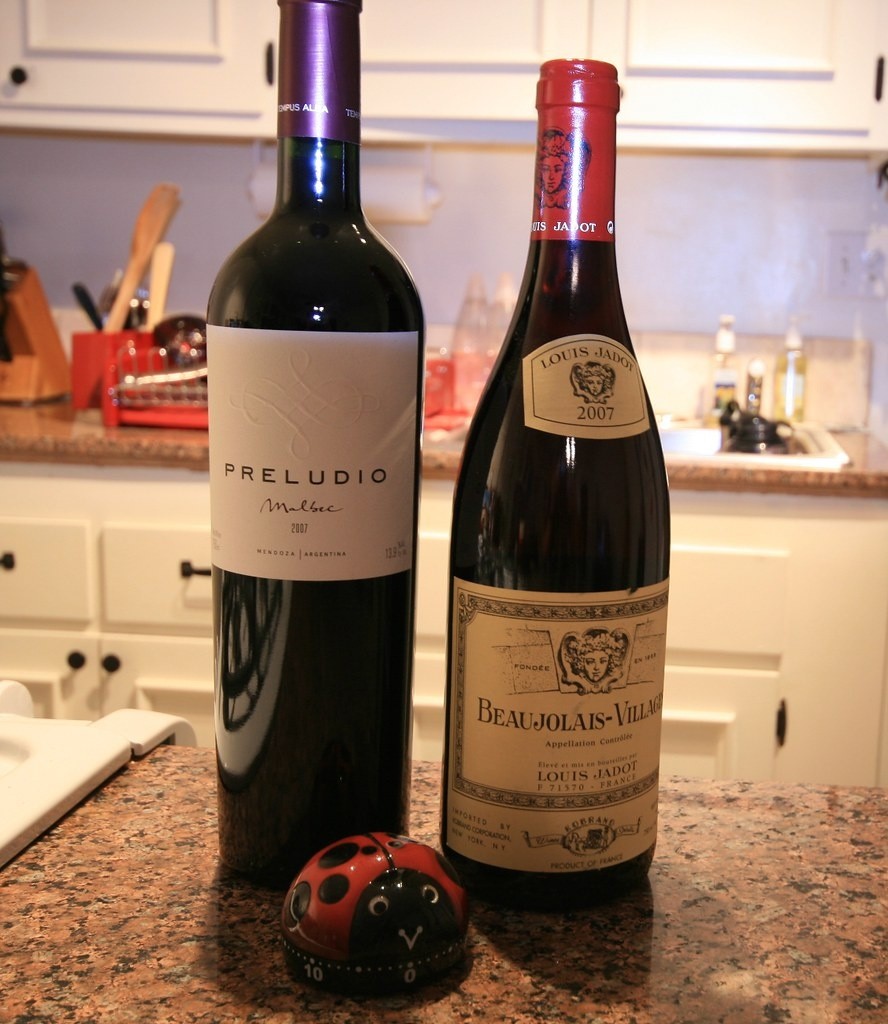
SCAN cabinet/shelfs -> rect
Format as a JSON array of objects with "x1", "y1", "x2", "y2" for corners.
[
  {"x1": 1, "y1": 1, "x2": 887, "y2": 161},
  {"x1": 1, "y1": 452, "x2": 888, "y2": 790}
]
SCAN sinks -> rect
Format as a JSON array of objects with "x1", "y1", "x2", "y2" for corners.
[{"x1": 662, "y1": 415, "x2": 853, "y2": 472}]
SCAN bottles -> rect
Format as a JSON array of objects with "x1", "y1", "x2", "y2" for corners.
[
  {"x1": 775, "y1": 318, "x2": 807, "y2": 425},
  {"x1": 704, "y1": 315, "x2": 736, "y2": 429},
  {"x1": 206, "y1": 0, "x2": 424, "y2": 887},
  {"x1": 488, "y1": 272, "x2": 518, "y2": 378},
  {"x1": 440, "y1": 58, "x2": 672, "y2": 911},
  {"x1": 451, "y1": 276, "x2": 490, "y2": 427}
]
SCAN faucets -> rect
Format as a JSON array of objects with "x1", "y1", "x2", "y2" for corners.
[{"x1": 745, "y1": 358, "x2": 767, "y2": 416}]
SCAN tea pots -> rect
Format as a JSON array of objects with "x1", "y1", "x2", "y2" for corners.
[{"x1": 722, "y1": 400, "x2": 794, "y2": 453}]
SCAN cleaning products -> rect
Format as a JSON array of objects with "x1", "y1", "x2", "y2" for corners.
[
  {"x1": 773, "y1": 312, "x2": 806, "y2": 420},
  {"x1": 709, "y1": 310, "x2": 737, "y2": 420}
]
